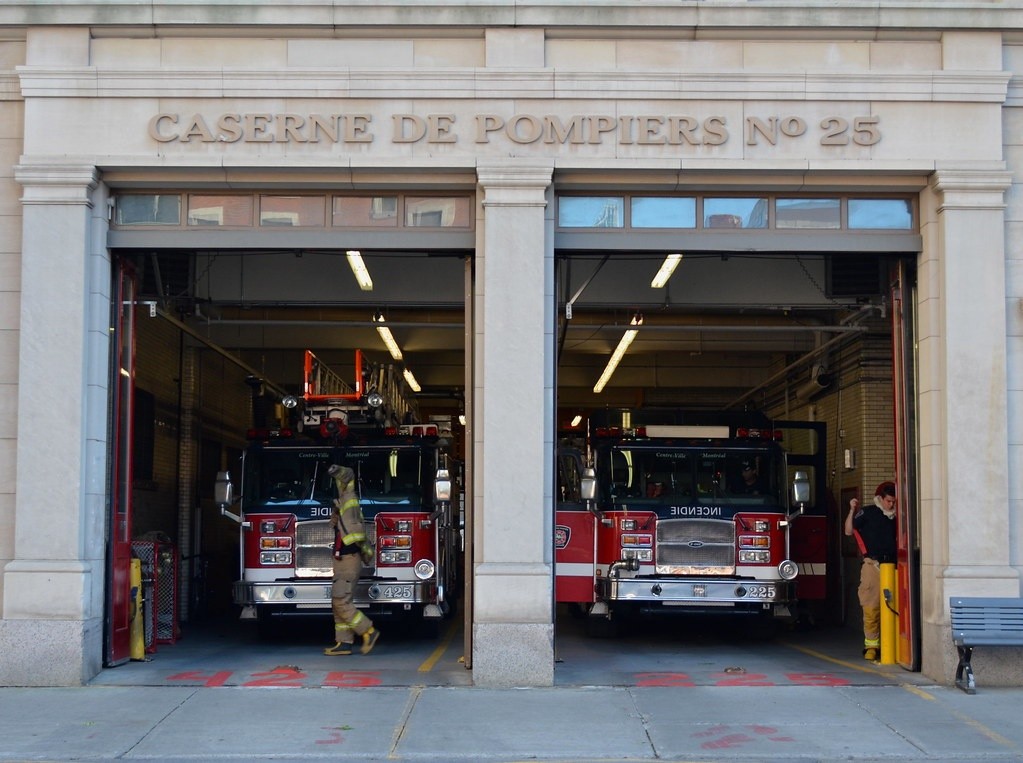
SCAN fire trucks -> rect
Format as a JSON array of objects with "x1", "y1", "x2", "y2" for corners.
[
  {"x1": 214, "y1": 345, "x2": 464, "y2": 636},
  {"x1": 554, "y1": 408, "x2": 829, "y2": 644}
]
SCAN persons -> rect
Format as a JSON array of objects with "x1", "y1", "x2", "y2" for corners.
[
  {"x1": 737, "y1": 455, "x2": 766, "y2": 498},
  {"x1": 325, "y1": 464, "x2": 382, "y2": 658},
  {"x1": 643, "y1": 465, "x2": 673, "y2": 498},
  {"x1": 844, "y1": 482, "x2": 900, "y2": 659}
]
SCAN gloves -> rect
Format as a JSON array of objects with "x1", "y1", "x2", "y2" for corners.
[{"x1": 335, "y1": 550, "x2": 342, "y2": 560}]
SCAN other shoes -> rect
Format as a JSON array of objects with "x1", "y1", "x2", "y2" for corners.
[
  {"x1": 865, "y1": 647, "x2": 876, "y2": 660},
  {"x1": 323, "y1": 641, "x2": 351, "y2": 655},
  {"x1": 360, "y1": 627, "x2": 379, "y2": 655}
]
